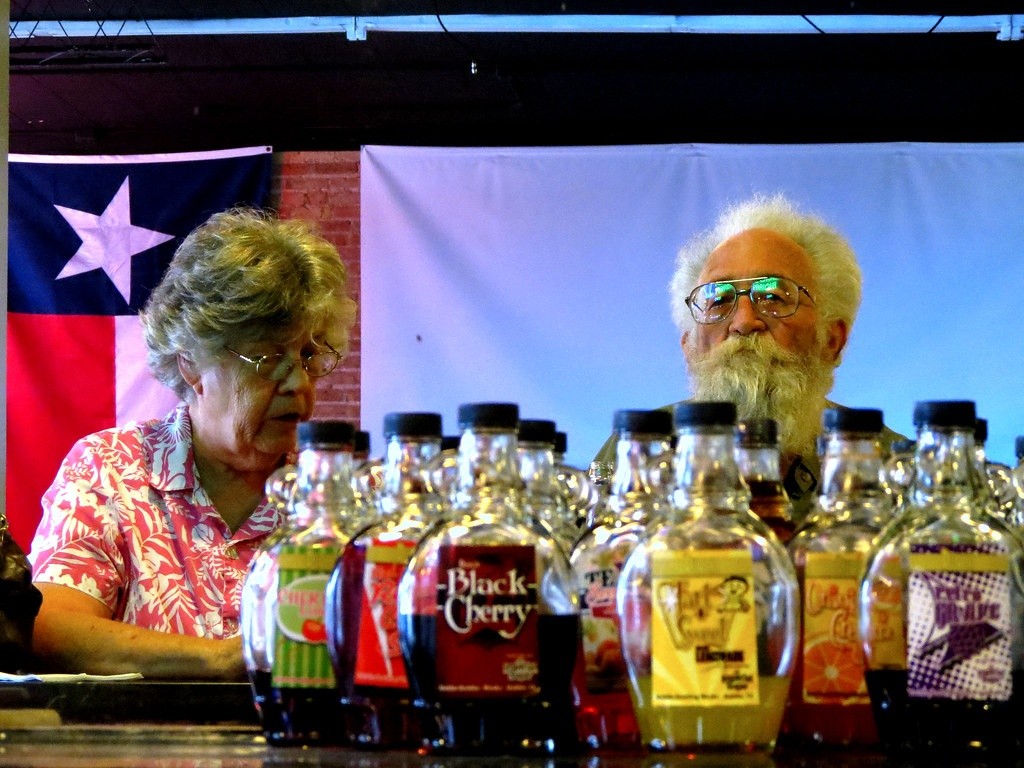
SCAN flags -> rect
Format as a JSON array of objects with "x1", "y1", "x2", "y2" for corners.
[{"x1": 4, "y1": 141, "x2": 274, "y2": 557}]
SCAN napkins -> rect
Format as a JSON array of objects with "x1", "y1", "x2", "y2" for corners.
[{"x1": 0, "y1": 672, "x2": 144, "y2": 683}]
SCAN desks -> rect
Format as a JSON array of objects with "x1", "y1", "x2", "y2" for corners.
[{"x1": 0, "y1": 671, "x2": 879, "y2": 767}]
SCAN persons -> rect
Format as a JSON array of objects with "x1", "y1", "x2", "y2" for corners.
[
  {"x1": 29, "y1": 207, "x2": 356, "y2": 681},
  {"x1": 579, "y1": 190, "x2": 916, "y2": 494}
]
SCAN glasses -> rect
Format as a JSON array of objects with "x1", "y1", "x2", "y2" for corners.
[
  {"x1": 222, "y1": 335, "x2": 345, "y2": 383},
  {"x1": 685, "y1": 275, "x2": 816, "y2": 325}
]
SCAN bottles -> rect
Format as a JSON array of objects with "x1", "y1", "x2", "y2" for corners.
[{"x1": 240, "y1": 398, "x2": 1024, "y2": 752}]
